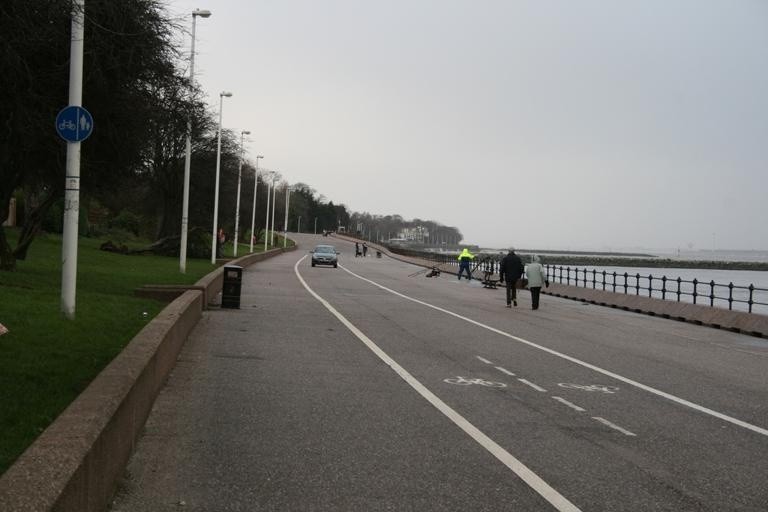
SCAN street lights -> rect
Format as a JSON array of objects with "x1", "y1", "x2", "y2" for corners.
[
  {"x1": 210, "y1": 89, "x2": 236, "y2": 263},
  {"x1": 178, "y1": 5, "x2": 212, "y2": 276},
  {"x1": 313, "y1": 216, "x2": 318, "y2": 236},
  {"x1": 297, "y1": 215, "x2": 303, "y2": 232},
  {"x1": 229, "y1": 124, "x2": 296, "y2": 258}
]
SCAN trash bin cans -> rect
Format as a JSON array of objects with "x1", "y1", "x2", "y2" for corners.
[{"x1": 221, "y1": 265, "x2": 243, "y2": 308}]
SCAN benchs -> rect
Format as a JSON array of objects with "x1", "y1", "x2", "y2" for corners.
[{"x1": 481, "y1": 270, "x2": 498, "y2": 289}]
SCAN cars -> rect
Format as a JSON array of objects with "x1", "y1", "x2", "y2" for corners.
[{"x1": 309, "y1": 244, "x2": 341, "y2": 269}]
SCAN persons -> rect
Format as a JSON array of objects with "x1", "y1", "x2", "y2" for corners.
[
  {"x1": 525, "y1": 254, "x2": 549, "y2": 310},
  {"x1": 362, "y1": 241, "x2": 368, "y2": 256},
  {"x1": 355, "y1": 241, "x2": 359, "y2": 256},
  {"x1": 457, "y1": 248, "x2": 477, "y2": 280},
  {"x1": 500, "y1": 246, "x2": 524, "y2": 306}
]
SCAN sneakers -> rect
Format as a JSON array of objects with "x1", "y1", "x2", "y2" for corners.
[
  {"x1": 513, "y1": 299, "x2": 517, "y2": 305},
  {"x1": 504, "y1": 303, "x2": 512, "y2": 308}
]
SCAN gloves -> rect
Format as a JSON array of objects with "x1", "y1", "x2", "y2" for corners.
[
  {"x1": 500, "y1": 277, "x2": 504, "y2": 283},
  {"x1": 545, "y1": 280, "x2": 549, "y2": 288}
]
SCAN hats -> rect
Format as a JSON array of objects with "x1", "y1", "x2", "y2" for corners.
[
  {"x1": 531, "y1": 253, "x2": 540, "y2": 263},
  {"x1": 508, "y1": 247, "x2": 515, "y2": 252}
]
